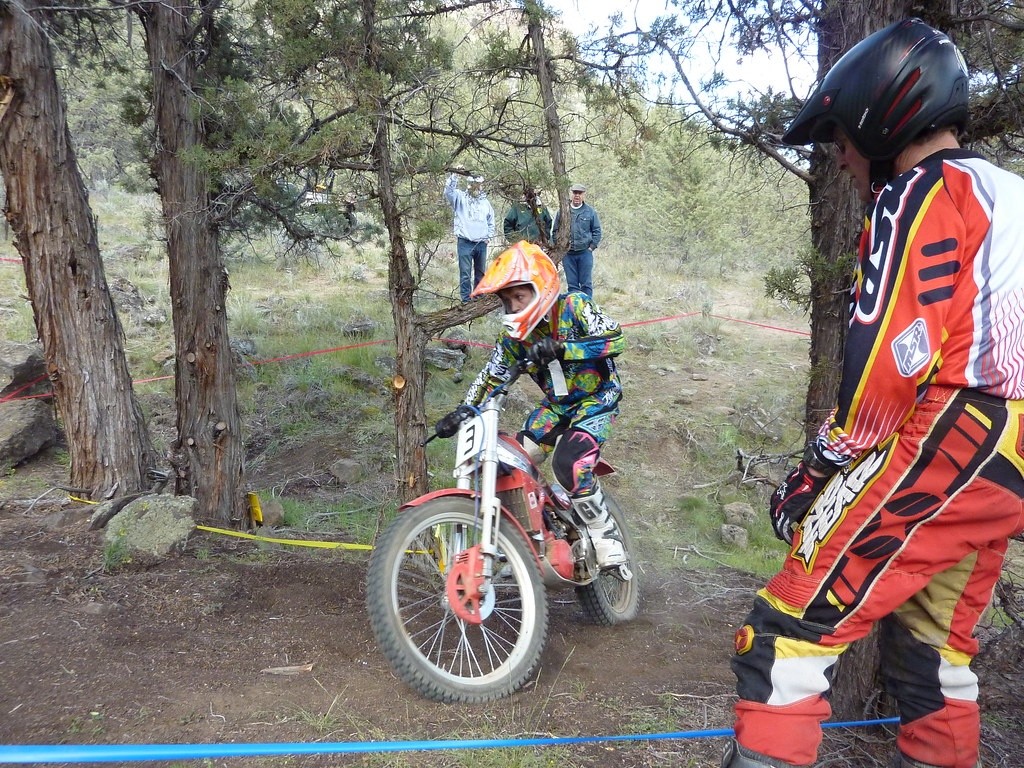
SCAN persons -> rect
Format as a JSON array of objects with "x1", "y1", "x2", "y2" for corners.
[
  {"x1": 726, "y1": 14, "x2": 1023, "y2": 768},
  {"x1": 445, "y1": 166, "x2": 603, "y2": 307},
  {"x1": 434, "y1": 240, "x2": 624, "y2": 580}
]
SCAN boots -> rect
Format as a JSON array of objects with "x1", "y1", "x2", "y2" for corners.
[{"x1": 567, "y1": 485, "x2": 627, "y2": 567}]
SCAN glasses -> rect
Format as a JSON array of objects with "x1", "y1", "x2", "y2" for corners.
[{"x1": 833, "y1": 137, "x2": 848, "y2": 155}]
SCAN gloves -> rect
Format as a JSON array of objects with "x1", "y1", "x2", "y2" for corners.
[
  {"x1": 435, "y1": 411, "x2": 459, "y2": 438},
  {"x1": 769, "y1": 459, "x2": 831, "y2": 546},
  {"x1": 527, "y1": 336, "x2": 565, "y2": 368}
]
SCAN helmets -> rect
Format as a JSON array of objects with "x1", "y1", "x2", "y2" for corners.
[
  {"x1": 469, "y1": 238, "x2": 562, "y2": 342},
  {"x1": 779, "y1": 15, "x2": 974, "y2": 161}
]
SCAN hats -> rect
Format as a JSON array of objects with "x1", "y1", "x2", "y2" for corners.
[
  {"x1": 467, "y1": 175, "x2": 484, "y2": 183},
  {"x1": 570, "y1": 184, "x2": 586, "y2": 192}
]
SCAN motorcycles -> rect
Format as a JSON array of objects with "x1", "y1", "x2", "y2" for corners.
[{"x1": 365, "y1": 344, "x2": 640, "y2": 705}]
